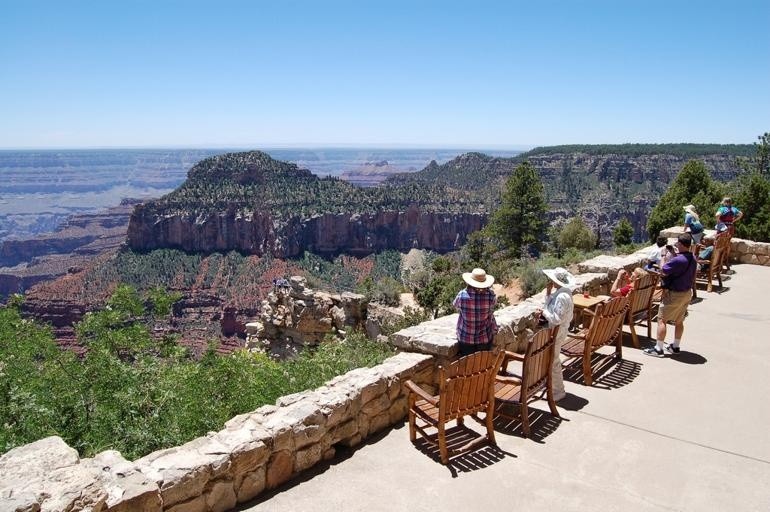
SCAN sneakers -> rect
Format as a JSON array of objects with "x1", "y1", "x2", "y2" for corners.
[
  {"x1": 665, "y1": 343, "x2": 681, "y2": 355},
  {"x1": 643, "y1": 346, "x2": 665, "y2": 358}
]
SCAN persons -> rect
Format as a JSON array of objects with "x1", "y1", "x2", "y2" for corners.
[
  {"x1": 534, "y1": 266, "x2": 578, "y2": 401},
  {"x1": 643, "y1": 234, "x2": 678, "y2": 274},
  {"x1": 679, "y1": 205, "x2": 705, "y2": 246},
  {"x1": 609, "y1": 267, "x2": 651, "y2": 326},
  {"x1": 694, "y1": 233, "x2": 716, "y2": 272},
  {"x1": 451, "y1": 267, "x2": 499, "y2": 357},
  {"x1": 642, "y1": 232, "x2": 698, "y2": 359},
  {"x1": 714, "y1": 197, "x2": 744, "y2": 266}
]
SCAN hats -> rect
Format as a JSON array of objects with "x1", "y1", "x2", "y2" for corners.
[
  {"x1": 682, "y1": 205, "x2": 700, "y2": 220},
  {"x1": 542, "y1": 267, "x2": 576, "y2": 289},
  {"x1": 462, "y1": 267, "x2": 495, "y2": 289}
]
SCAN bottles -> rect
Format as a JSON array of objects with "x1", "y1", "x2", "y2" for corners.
[{"x1": 584, "y1": 282, "x2": 589, "y2": 299}]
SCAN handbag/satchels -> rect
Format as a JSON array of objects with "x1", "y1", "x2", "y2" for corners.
[{"x1": 689, "y1": 222, "x2": 704, "y2": 234}]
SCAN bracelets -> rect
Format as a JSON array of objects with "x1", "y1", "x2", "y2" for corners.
[{"x1": 661, "y1": 254, "x2": 667, "y2": 257}]
